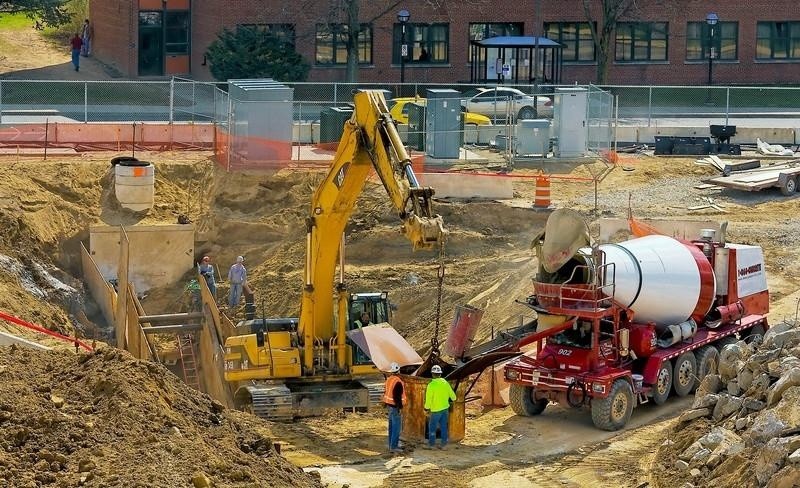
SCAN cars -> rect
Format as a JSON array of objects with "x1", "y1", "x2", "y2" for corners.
[{"x1": 459, "y1": 85, "x2": 553, "y2": 119}]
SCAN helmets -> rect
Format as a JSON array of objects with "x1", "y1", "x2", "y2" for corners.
[
  {"x1": 391, "y1": 362, "x2": 400, "y2": 373},
  {"x1": 430, "y1": 365, "x2": 443, "y2": 374}
]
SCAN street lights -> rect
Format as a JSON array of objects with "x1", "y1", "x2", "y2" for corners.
[
  {"x1": 396, "y1": 8, "x2": 411, "y2": 83},
  {"x1": 703, "y1": 13, "x2": 719, "y2": 106}
]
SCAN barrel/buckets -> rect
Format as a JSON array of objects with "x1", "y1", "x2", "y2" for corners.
[
  {"x1": 115, "y1": 163, "x2": 154, "y2": 212},
  {"x1": 444, "y1": 302, "x2": 483, "y2": 357}
]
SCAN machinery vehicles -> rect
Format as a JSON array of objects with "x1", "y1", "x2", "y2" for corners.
[
  {"x1": 222, "y1": 88, "x2": 453, "y2": 423},
  {"x1": 411, "y1": 221, "x2": 771, "y2": 433}
]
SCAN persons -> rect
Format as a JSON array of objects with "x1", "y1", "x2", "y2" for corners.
[
  {"x1": 570, "y1": 321, "x2": 592, "y2": 348},
  {"x1": 69, "y1": 32, "x2": 84, "y2": 73},
  {"x1": 228, "y1": 255, "x2": 249, "y2": 309},
  {"x1": 418, "y1": 48, "x2": 429, "y2": 62},
  {"x1": 381, "y1": 362, "x2": 406, "y2": 453},
  {"x1": 80, "y1": 18, "x2": 92, "y2": 57},
  {"x1": 199, "y1": 256, "x2": 217, "y2": 304},
  {"x1": 422, "y1": 364, "x2": 457, "y2": 451},
  {"x1": 354, "y1": 312, "x2": 371, "y2": 328}
]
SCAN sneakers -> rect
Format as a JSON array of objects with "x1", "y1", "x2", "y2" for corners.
[
  {"x1": 422, "y1": 445, "x2": 448, "y2": 450},
  {"x1": 389, "y1": 444, "x2": 403, "y2": 452}
]
